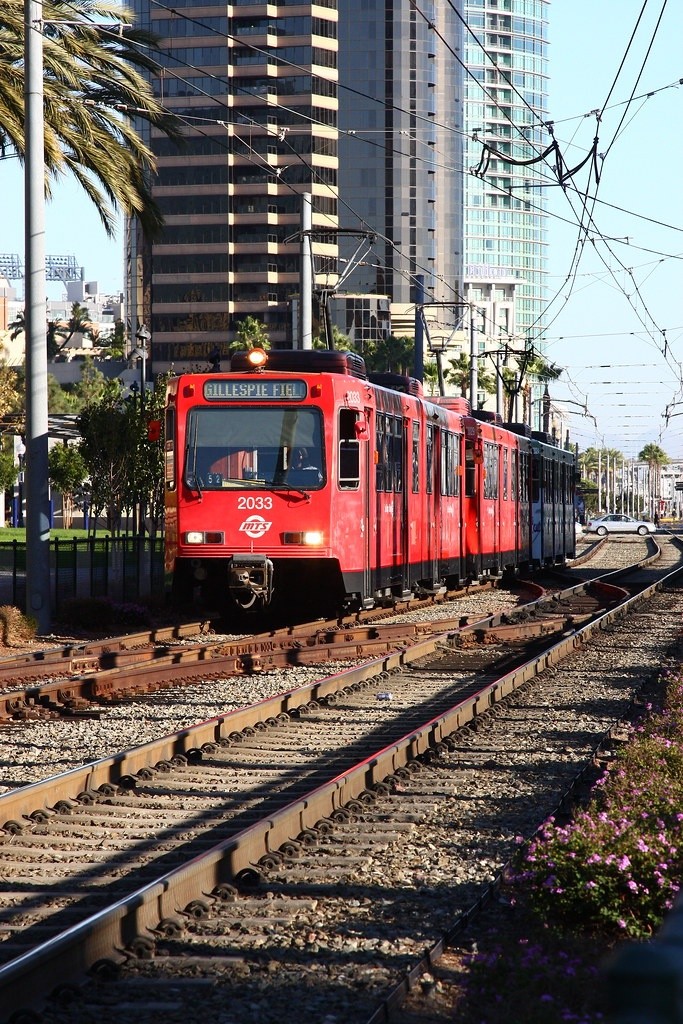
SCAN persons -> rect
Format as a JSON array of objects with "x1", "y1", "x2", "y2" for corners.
[
  {"x1": 654, "y1": 511, "x2": 661, "y2": 528},
  {"x1": 289, "y1": 447, "x2": 324, "y2": 485}
]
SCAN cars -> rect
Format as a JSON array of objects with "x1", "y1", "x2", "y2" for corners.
[{"x1": 585, "y1": 513, "x2": 657, "y2": 536}]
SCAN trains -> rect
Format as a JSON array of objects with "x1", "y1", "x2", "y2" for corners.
[{"x1": 152, "y1": 343, "x2": 579, "y2": 620}]
